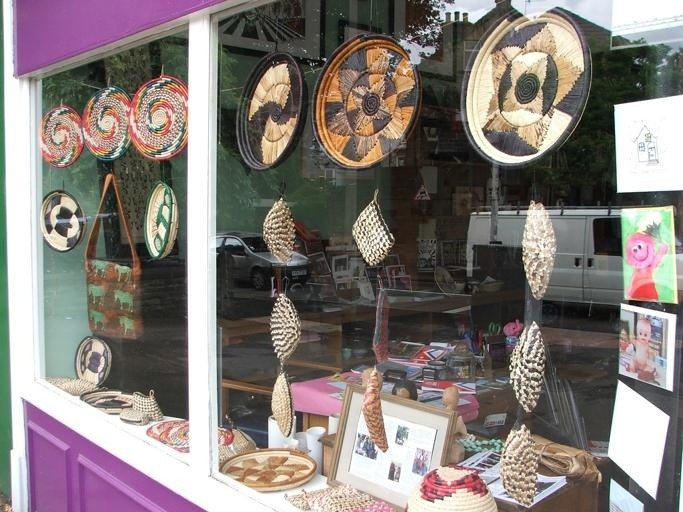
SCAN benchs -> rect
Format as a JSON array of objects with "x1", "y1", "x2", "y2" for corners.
[{"x1": 219, "y1": 377, "x2": 274, "y2": 423}]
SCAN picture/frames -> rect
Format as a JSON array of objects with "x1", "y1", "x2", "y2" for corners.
[{"x1": 328, "y1": 381, "x2": 459, "y2": 509}]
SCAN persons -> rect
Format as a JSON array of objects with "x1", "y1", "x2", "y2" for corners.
[{"x1": 628, "y1": 318, "x2": 657, "y2": 375}]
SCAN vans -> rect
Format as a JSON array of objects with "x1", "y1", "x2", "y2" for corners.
[{"x1": 466, "y1": 205, "x2": 682, "y2": 327}]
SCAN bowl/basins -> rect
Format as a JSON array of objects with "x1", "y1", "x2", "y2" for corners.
[{"x1": 482, "y1": 280, "x2": 504, "y2": 293}]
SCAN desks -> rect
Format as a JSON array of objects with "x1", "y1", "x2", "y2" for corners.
[
  {"x1": 290, "y1": 366, "x2": 516, "y2": 431},
  {"x1": 220, "y1": 288, "x2": 524, "y2": 379}
]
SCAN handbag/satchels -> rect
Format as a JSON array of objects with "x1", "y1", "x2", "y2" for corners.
[
  {"x1": 499, "y1": 419, "x2": 538, "y2": 508},
  {"x1": 120, "y1": 390, "x2": 163, "y2": 426},
  {"x1": 270, "y1": 293, "x2": 302, "y2": 362},
  {"x1": 361, "y1": 370, "x2": 388, "y2": 453},
  {"x1": 262, "y1": 198, "x2": 295, "y2": 264},
  {"x1": 521, "y1": 200, "x2": 557, "y2": 300},
  {"x1": 373, "y1": 289, "x2": 390, "y2": 364},
  {"x1": 86, "y1": 259, "x2": 143, "y2": 340},
  {"x1": 509, "y1": 322, "x2": 546, "y2": 414},
  {"x1": 272, "y1": 371, "x2": 293, "y2": 438},
  {"x1": 352, "y1": 200, "x2": 396, "y2": 266}
]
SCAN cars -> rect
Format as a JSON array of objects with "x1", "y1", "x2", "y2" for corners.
[{"x1": 216, "y1": 231, "x2": 311, "y2": 290}]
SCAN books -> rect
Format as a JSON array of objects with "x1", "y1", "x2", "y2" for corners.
[{"x1": 349, "y1": 340, "x2": 568, "y2": 510}]
